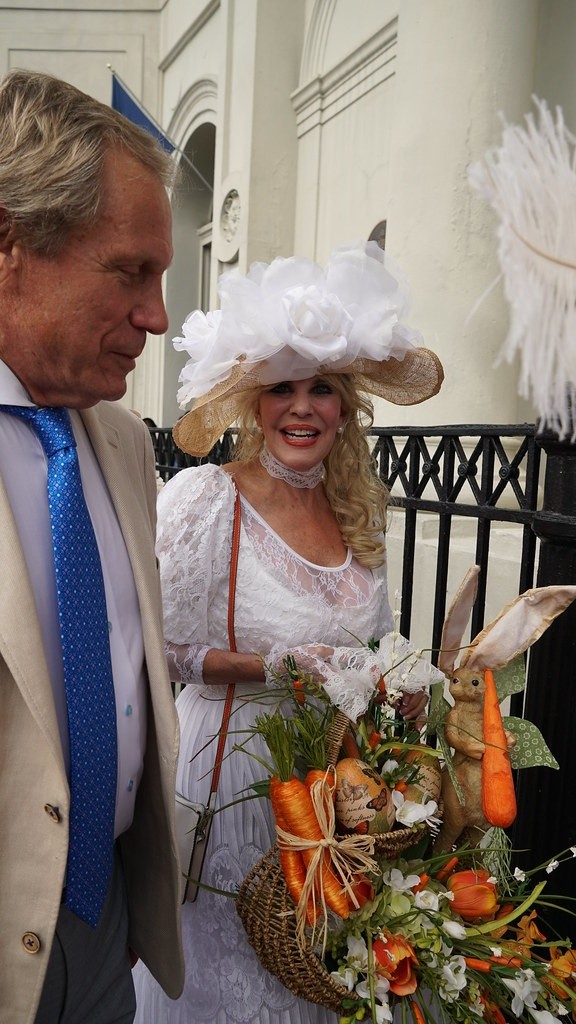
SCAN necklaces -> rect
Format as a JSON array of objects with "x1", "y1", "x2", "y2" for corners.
[{"x1": 259, "y1": 440, "x2": 326, "y2": 488}]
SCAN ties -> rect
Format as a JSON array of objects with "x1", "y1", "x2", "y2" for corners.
[{"x1": 0, "y1": 405, "x2": 119, "y2": 929}]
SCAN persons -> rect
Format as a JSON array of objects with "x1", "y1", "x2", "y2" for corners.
[
  {"x1": 133, "y1": 236, "x2": 444, "y2": 1024},
  {"x1": 0, "y1": 68, "x2": 189, "y2": 1023}
]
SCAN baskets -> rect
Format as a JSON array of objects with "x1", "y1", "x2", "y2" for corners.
[{"x1": 235, "y1": 689, "x2": 425, "y2": 1017}]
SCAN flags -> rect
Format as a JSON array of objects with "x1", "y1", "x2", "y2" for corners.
[{"x1": 111, "y1": 72, "x2": 175, "y2": 158}]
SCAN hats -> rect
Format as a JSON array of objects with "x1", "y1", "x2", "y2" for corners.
[{"x1": 172, "y1": 247, "x2": 444, "y2": 457}]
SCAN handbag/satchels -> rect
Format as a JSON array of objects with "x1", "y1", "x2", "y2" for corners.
[{"x1": 173, "y1": 791, "x2": 212, "y2": 906}]
